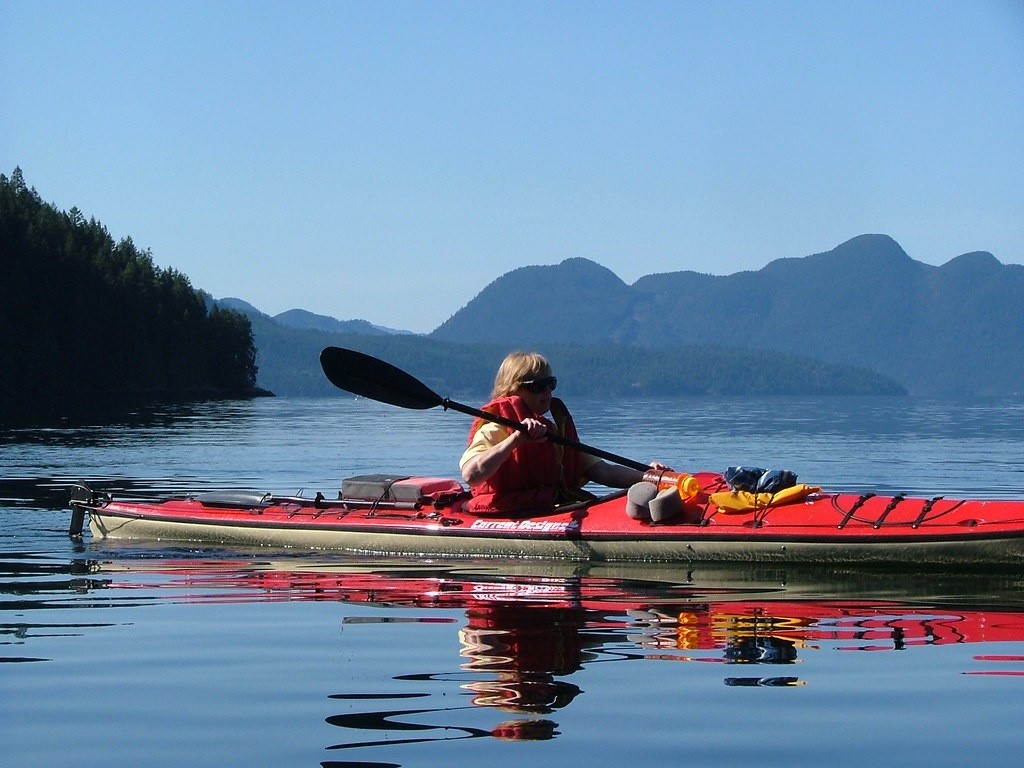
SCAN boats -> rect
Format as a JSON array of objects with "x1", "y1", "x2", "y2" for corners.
[{"x1": 59, "y1": 470, "x2": 1024, "y2": 578}]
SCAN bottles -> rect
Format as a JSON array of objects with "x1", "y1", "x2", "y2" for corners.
[{"x1": 642, "y1": 470, "x2": 697, "y2": 503}]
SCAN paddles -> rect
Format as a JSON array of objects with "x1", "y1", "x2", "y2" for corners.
[{"x1": 318, "y1": 343, "x2": 658, "y2": 473}]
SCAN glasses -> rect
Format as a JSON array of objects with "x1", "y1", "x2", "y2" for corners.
[{"x1": 519, "y1": 376, "x2": 557, "y2": 393}]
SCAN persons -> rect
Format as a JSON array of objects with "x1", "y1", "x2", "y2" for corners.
[
  {"x1": 459, "y1": 353, "x2": 674, "y2": 512},
  {"x1": 456, "y1": 606, "x2": 674, "y2": 740}
]
